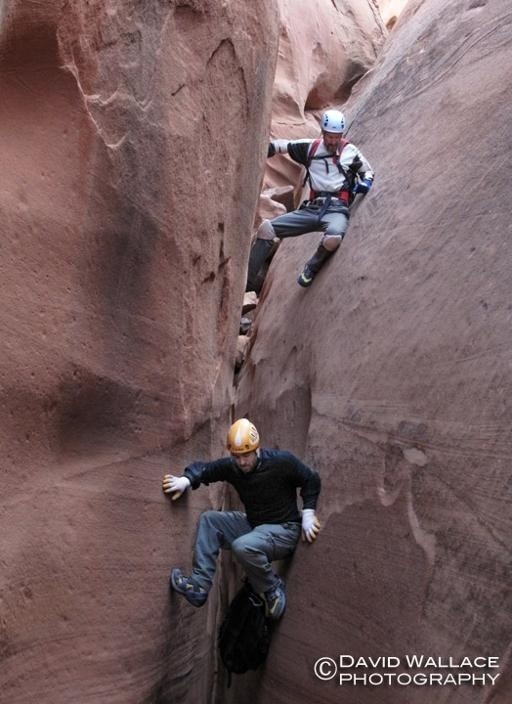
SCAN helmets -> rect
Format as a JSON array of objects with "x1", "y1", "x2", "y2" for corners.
[
  {"x1": 320, "y1": 110, "x2": 346, "y2": 133},
  {"x1": 226, "y1": 418, "x2": 260, "y2": 456}
]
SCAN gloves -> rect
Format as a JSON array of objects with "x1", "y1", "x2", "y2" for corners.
[
  {"x1": 163, "y1": 475, "x2": 191, "y2": 500},
  {"x1": 301, "y1": 509, "x2": 320, "y2": 543},
  {"x1": 353, "y1": 178, "x2": 372, "y2": 194}
]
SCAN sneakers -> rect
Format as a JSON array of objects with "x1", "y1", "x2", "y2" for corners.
[
  {"x1": 298, "y1": 262, "x2": 315, "y2": 287},
  {"x1": 171, "y1": 569, "x2": 208, "y2": 608},
  {"x1": 264, "y1": 573, "x2": 286, "y2": 620}
]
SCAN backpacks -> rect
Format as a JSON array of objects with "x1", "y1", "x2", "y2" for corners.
[{"x1": 217, "y1": 583, "x2": 271, "y2": 688}]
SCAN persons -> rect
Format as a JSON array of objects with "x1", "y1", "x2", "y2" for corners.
[
  {"x1": 161, "y1": 418, "x2": 322, "y2": 621},
  {"x1": 242, "y1": 110, "x2": 375, "y2": 317}
]
